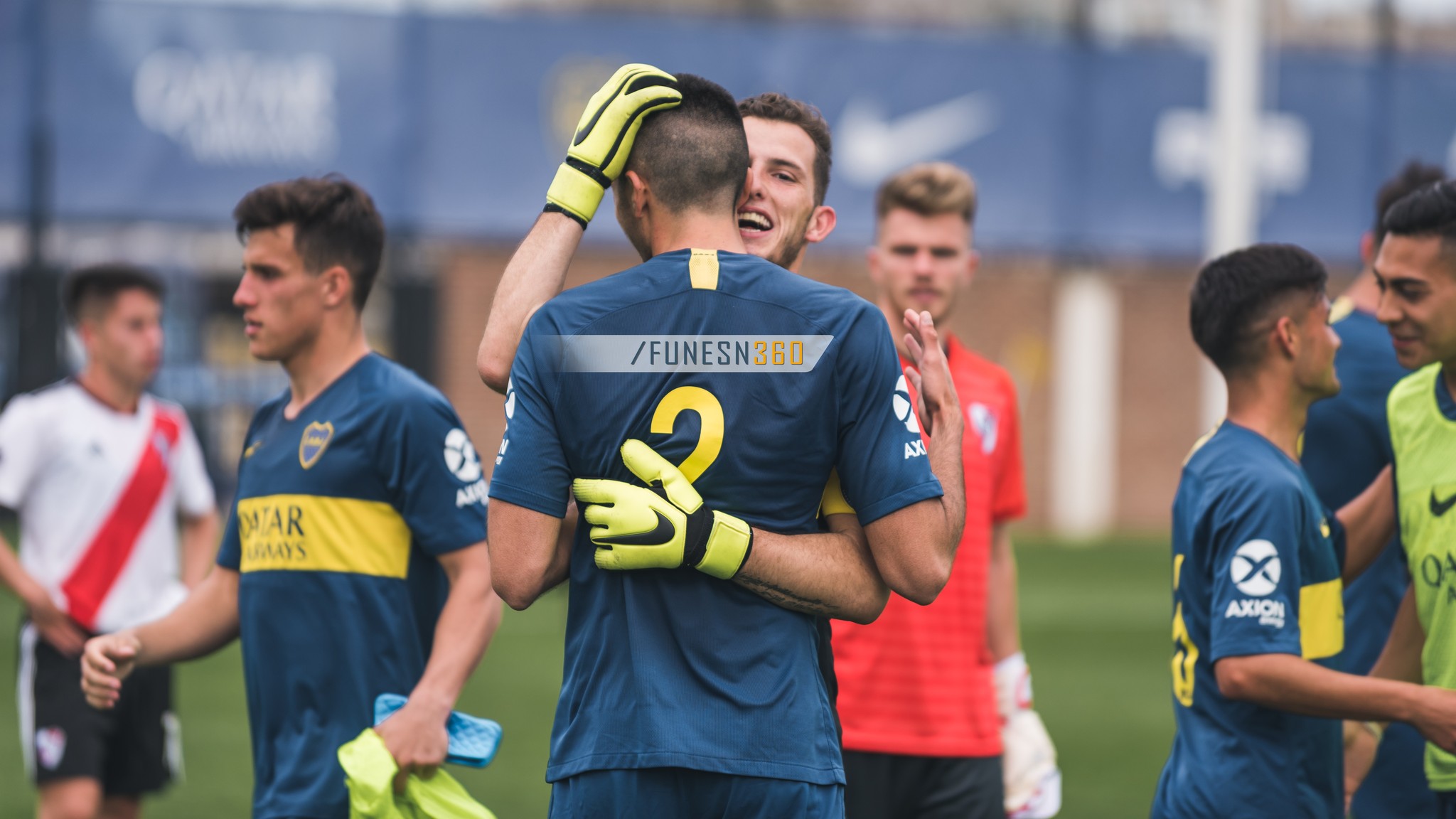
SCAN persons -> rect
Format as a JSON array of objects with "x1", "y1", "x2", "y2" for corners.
[
  {"x1": 829, "y1": 152, "x2": 1063, "y2": 819},
  {"x1": 1342, "y1": 173, "x2": 1456, "y2": 819},
  {"x1": 78, "y1": 171, "x2": 501, "y2": 819},
  {"x1": 0, "y1": 262, "x2": 224, "y2": 819},
  {"x1": 474, "y1": 49, "x2": 965, "y2": 819},
  {"x1": 1148, "y1": 239, "x2": 1456, "y2": 819},
  {"x1": 1296, "y1": 161, "x2": 1447, "y2": 819}
]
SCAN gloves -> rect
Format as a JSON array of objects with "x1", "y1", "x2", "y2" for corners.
[
  {"x1": 573, "y1": 436, "x2": 754, "y2": 591},
  {"x1": 995, "y1": 654, "x2": 1065, "y2": 819},
  {"x1": 543, "y1": 63, "x2": 684, "y2": 227}
]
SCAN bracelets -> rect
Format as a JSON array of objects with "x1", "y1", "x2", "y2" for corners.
[{"x1": 1362, "y1": 722, "x2": 1385, "y2": 741}]
